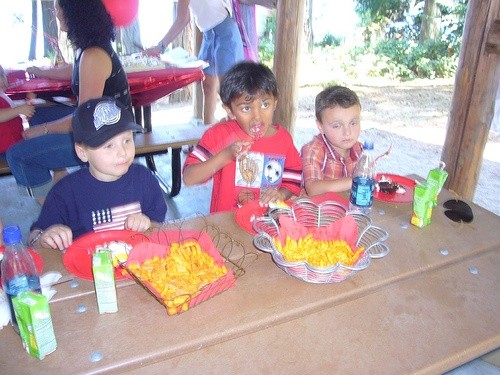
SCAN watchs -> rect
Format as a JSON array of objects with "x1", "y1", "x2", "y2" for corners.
[{"x1": 159, "y1": 40, "x2": 166, "y2": 49}]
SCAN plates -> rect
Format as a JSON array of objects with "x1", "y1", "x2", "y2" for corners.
[
  {"x1": 235, "y1": 199, "x2": 296, "y2": 234},
  {"x1": 372, "y1": 173, "x2": 418, "y2": 203},
  {"x1": 63, "y1": 229, "x2": 150, "y2": 281}
]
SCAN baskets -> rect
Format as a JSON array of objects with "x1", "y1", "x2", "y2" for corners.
[
  {"x1": 252, "y1": 199, "x2": 390, "y2": 283},
  {"x1": 118, "y1": 215, "x2": 258, "y2": 316}
]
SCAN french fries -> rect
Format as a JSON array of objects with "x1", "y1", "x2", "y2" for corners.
[
  {"x1": 271, "y1": 234, "x2": 365, "y2": 269},
  {"x1": 113, "y1": 241, "x2": 228, "y2": 314}
]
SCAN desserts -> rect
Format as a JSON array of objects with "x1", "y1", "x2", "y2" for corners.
[{"x1": 374, "y1": 175, "x2": 400, "y2": 194}]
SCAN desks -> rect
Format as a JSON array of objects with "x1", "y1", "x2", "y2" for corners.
[
  {"x1": 2, "y1": 53, "x2": 206, "y2": 134},
  {"x1": 0, "y1": 174, "x2": 500, "y2": 375}
]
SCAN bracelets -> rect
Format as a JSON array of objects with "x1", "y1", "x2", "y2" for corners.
[
  {"x1": 43, "y1": 121, "x2": 49, "y2": 135},
  {"x1": 31, "y1": 231, "x2": 42, "y2": 249}
]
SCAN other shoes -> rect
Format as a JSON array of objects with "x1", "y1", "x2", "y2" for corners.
[{"x1": 183, "y1": 145, "x2": 194, "y2": 155}]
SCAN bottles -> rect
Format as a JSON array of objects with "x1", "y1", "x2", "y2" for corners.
[
  {"x1": 348, "y1": 140, "x2": 375, "y2": 217},
  {"x1": 0, "y1": 224, "x2": 42, "y2": 336}
]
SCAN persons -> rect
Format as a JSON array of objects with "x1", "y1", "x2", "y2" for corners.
[
  {"x1": 301, "y1": 86, "x2": 374, "y2": 196},
  {"x1": 28, "y1": 97, "x2": 167, "y2": 251},
  {"x1": 0, "y1": 64, "x2": 37, "y2": 160},
  {"x1": 182, "y1": 62, "x2": 303, "y2": 214},
  {"x1": 144, "y1": 0, "x2": 245, "y2": 126},
  {"x1": 6, "y1": 0, "x2": 135, "y2": 207}
]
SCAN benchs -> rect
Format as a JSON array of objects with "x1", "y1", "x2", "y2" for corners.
[{"x1": 0, "y1": 125, "x2": 212, "y2": 197}]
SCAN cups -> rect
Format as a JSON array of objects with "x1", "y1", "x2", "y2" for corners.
[
  {"x1": 25, "y1": 61, "x2": 35, "y2": 82},
  {"x1": 140, "y1": 55, "x2": 159, "y2": 67}
]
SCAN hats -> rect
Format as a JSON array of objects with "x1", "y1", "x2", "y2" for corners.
[{"x1": 72, "y1": 97, "x2": 147, "y2": 147}]
